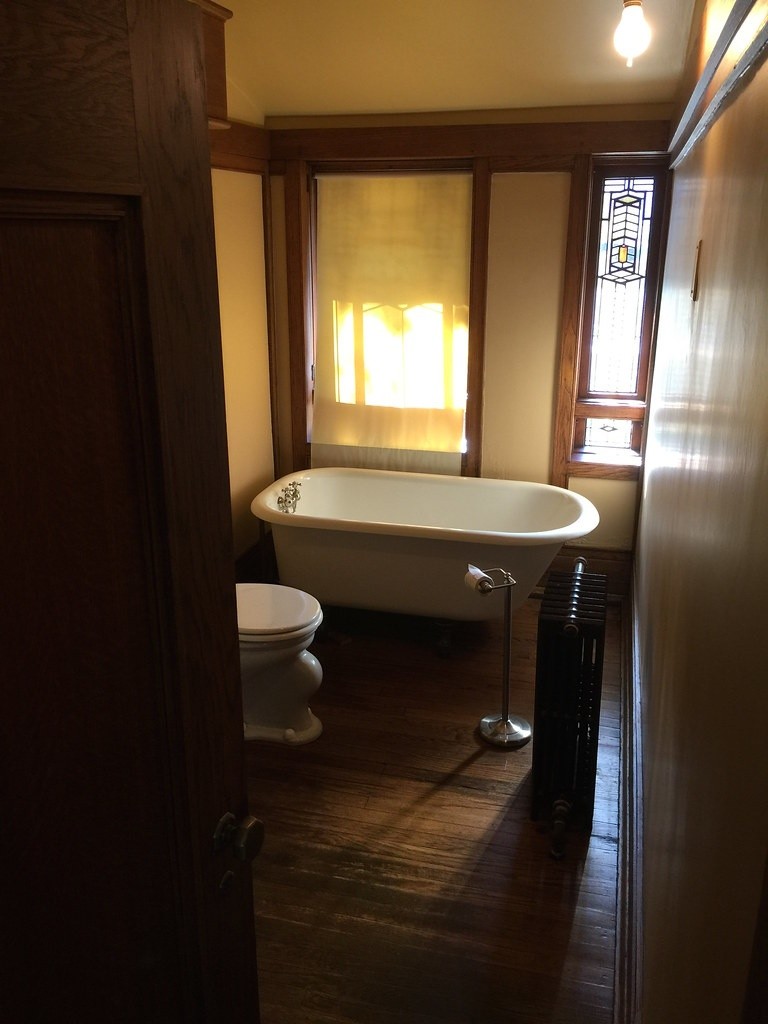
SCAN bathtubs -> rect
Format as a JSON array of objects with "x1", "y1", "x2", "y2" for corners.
[{"x1": 250, "y1": 464, "x2": 601, "y2": 623}]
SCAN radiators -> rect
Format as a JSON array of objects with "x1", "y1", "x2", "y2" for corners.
[{"x1": 531, "y1": 556, "x2": 609, "y2": 856}]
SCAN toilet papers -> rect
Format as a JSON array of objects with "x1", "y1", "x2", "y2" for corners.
[{"x1": 462, "y1": 562, "x2": 493, "y2": 597}]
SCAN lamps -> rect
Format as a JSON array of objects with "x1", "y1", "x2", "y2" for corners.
[{"x1": 613, "y1": 0, "x2": 652, "y2": 60}]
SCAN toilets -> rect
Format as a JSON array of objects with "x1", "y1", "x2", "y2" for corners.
[{"x1": 235, "y1": 578, "x2": 328, "y2": 747}]
